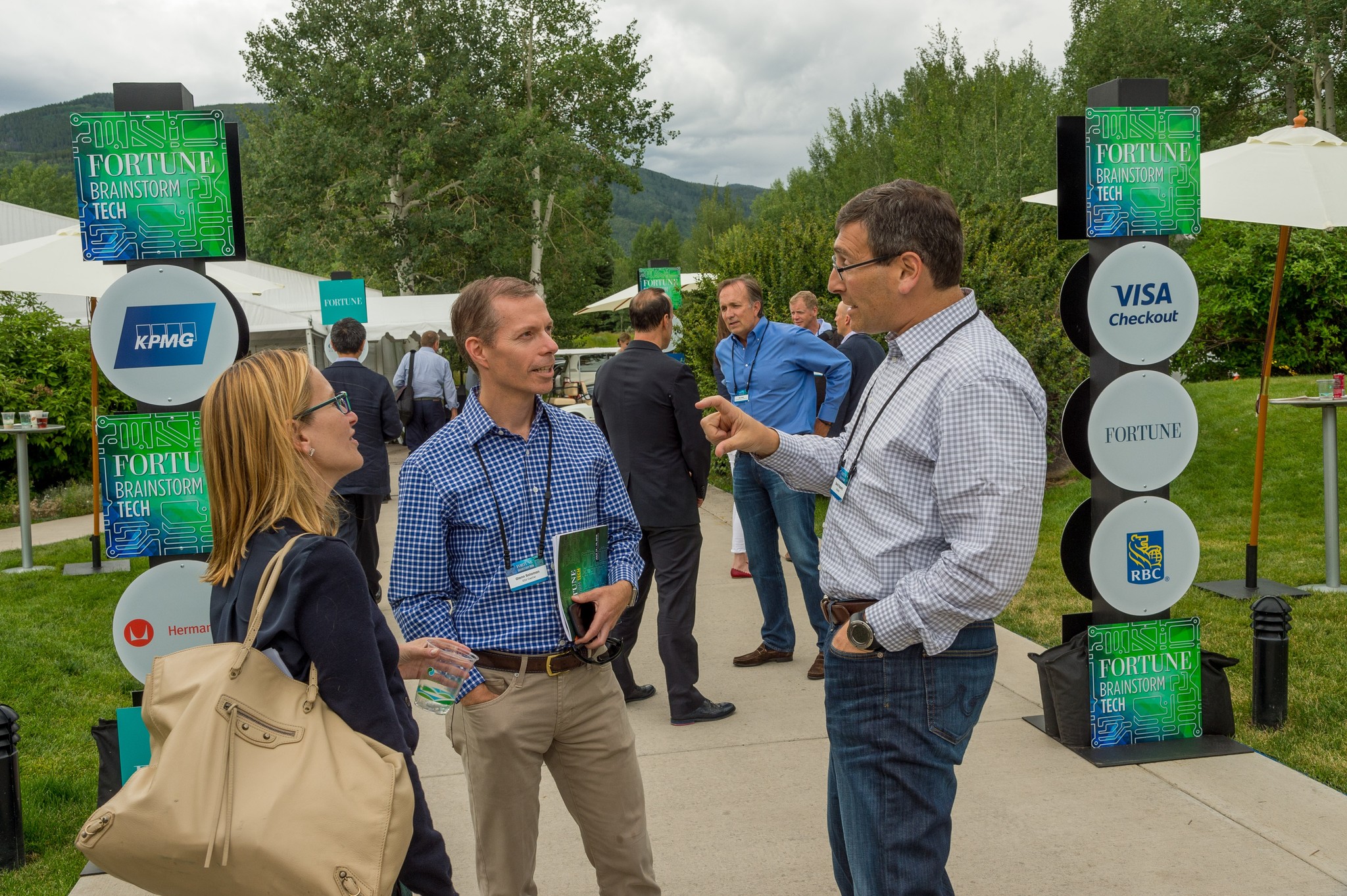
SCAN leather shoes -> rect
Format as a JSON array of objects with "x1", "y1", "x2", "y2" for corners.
[
  {"x1": 623, "y1": 685, "x2": 655, "y2": 703},
  {"x1": 732, "y1": 644, "x2": 793, "y2": 667},
  {"x1": 807, "y1": 652, "x2": 826, "y2": 679},
  {"x1": 669, "y1": 698, "x2": 736, "y2": 727},
  {"x1": 375, "y1": 585, "x2": 382, "y2": 605}
]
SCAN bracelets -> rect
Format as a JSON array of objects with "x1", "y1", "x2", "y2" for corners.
[{"x1": 818, "y1": 419, "x2": 833, "y2": 426}]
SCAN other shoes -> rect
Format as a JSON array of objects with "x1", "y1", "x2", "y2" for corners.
[
  {"x1": 785, "y1": 552, "x2": 792, "y2": 561},
  {"x1": 731, "y1": 567, "x2": 752, "y2": 578}
]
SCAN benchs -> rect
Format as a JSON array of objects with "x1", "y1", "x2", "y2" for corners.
[
  {"x1": 578, "y1": 380, "x2": 594, "y2": 405},
  {"x1": 546, "y1": 383, "x2": 577, "y2": 406}
]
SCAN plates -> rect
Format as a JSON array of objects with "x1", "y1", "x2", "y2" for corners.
[{"x1": 1341, "y1": 396, "x2": 1347, "y2": 398}]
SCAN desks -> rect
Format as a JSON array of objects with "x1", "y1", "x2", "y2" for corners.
[
  {"x1": 0, "y1": 422, "x2": 67, "y2": 574},
  {"x1": 1269, "y1": 392, "x2": 1347, "y2": 592}
]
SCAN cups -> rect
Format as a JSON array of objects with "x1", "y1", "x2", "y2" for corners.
[
  {"x1": 1316, "y1": 379, "x2": 1335, "y2": 401},
  {"x1": 29, "y1": 410, "x2": 43, "y2": 427},
  {"x1": 414, "y1": 639, "x2": 479, "y2": 715},
  {"x1": 35, "y1": 412, "x2": 49, "y2": 428},
  {"x1": 1, "y1": 412, "x2": 15, "y2": 428},
  {"x1": 19, "y1": 412, "x2": 32, "y2": 428}
]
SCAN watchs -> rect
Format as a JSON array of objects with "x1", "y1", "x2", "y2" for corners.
[
  {"x1": 627, "y1": 583, "x2": 638, "y2": 606},
  {"x1": 846, "y1": 609, "x2": 882, "y2": 651}
]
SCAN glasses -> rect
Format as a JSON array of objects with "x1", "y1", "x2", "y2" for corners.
[
  {"x1": 294, "y1": 391, "x2": 351, "y2": 423},
  {"x1": 832, "y1": 254, "x2": 926, "y2": 281}
]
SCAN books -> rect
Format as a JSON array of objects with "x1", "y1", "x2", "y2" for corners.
[{"x1": 552, "y1": 524, "x2": 608, "y2": 642}]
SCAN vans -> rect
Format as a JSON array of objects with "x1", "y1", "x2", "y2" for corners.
[{"x1": 549, "y1": 352, "x2": 617, "y2": 404}]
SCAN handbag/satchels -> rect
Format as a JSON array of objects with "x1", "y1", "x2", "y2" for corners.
[
  {"x1": 395, "y1": 385, "x2": 414, "y2": 427},
  {"x1": 76, "y1": 535, "x2": 415, "y2": 895}
]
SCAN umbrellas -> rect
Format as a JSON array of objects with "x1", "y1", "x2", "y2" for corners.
[
  {"x1": 1018, "y1": 111, "x2": 1347, "y2": 546},
  {"x1": 573, "y1": 273, "x2": 721, "y2": 316},
  {"x1": 0, "y1": 227, "x2": 125, "y2": 535}
]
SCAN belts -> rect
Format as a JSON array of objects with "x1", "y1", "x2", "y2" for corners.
[
  {"x1": 414, "y1": 396, "x2": 441, "y2": 401},
  {"x1": 470, "y1": 647, "x2": 602, "y2": 681},
  {"x1": 819, "y1": 595, "x2": 881, "y2": 624}
]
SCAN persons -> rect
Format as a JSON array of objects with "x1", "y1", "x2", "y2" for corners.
[
  {"x1": 392, "y1": 331, "x2": 457, "y2": 455},
  {"x1": 592, "y1": 288, "x2": 737, "y2": 726},
  {"x1": 386, "y1": 278, "x2": 663, "y2": 896},
  {"x1": 615, "y1": 332, "x2": 631, "y2": 354},
  {"x1": 201, "y1": 348, "x2": 457, "y2": 896},
  {"x1": 320, "y1": 318, "x2": 402, "y2": 605},
  {"x1": 693, "y1": 179, "x2": 1048, "y2": 896}
]
